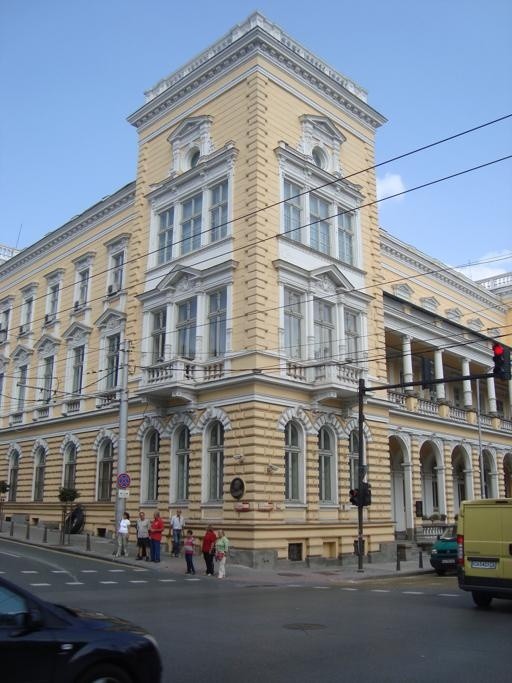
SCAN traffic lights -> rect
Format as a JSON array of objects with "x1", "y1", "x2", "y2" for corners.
[
  {"x1": 349, "y1": 488, "x2": 360, "y2": 505},
  {"x1": 366, "y1": 490, "x2": 371, "y2": 505},
  {"x1": 492, "y1": 343, "x2": 512, "y2": 379}
]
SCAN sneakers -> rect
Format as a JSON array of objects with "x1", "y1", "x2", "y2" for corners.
[
  {"x1": 218, "y1": 575, "x2": 225, "y2": 578},
  {"x1": 124, "y1": 554, "x2": 129, "y2": 557},
  {"x1": 116, "y1": 553, "x2": 121, "y2": 557},
  {"x1": 203, "y1": 572, "x2": 211, "y2": 576},
  {"x1": 185, "y1": 572, "x2": 195, "y2": 574}
]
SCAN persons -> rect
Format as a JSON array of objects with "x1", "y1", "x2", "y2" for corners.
[{"x1": 116, "y1": 509, "x2": 229, "y2": 578}]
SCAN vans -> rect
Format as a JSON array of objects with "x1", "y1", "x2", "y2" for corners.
[{"x1": 430, "y1": 523, "x2": 458, "y2": 576}]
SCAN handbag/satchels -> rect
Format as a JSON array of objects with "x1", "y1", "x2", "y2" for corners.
[{"x1": 215, "y1": 552, "x2": 223, "y2": 560}]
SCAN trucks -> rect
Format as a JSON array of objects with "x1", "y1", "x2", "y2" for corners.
[{"x1": 457, "y1": 498, "x2": 512, "y2": 610}]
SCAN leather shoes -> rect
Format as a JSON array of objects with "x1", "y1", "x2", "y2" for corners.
[{"x1": 149, "y1": 560, "x2": 161, "y2": 562}]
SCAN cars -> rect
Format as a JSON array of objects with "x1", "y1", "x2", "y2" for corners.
[{"x1": 0, "y1": 576, "x2": 162, "y2": 682}]
